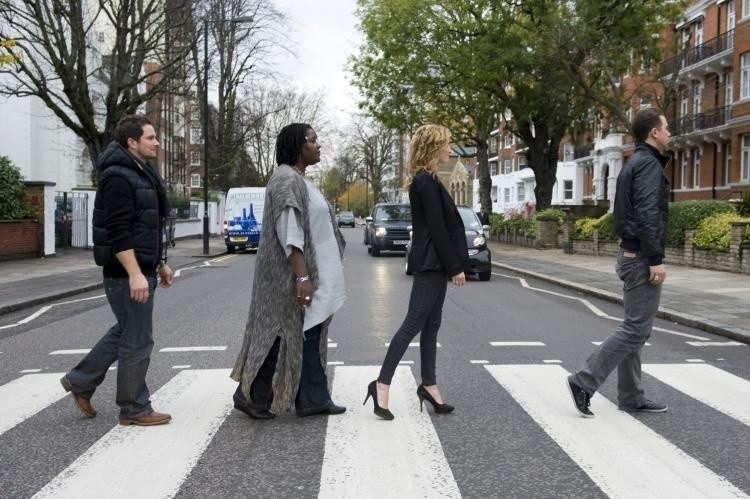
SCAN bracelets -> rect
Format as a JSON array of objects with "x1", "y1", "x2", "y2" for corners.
[{"x1": 294, "y1": 275, "x2": 310, "y2": 284}]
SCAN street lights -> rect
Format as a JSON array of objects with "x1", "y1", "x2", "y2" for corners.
[{"x1": 202, "y1": 15, "x2": 255, "y2": 256}]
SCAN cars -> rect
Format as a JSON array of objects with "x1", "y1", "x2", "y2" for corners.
[{"x1": 405, "y1": 203, "x2": 493, "y2": 282}]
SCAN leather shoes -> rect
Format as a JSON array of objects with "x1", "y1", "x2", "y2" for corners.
[
  {"x1": 60, "y1": 373, "x2": 97, "y2": 418},
  {"x1": 232, "y1": 393, "x2": 277, "y2": 420},
  {"x1": 118, "y1": 409, "x2": 172, "y2": 426},
  {"x1": 295, "y1": 400, "x2": 347, "y2": 417}
]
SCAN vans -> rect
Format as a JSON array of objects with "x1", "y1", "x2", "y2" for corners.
[{"x1": 222, "y1": 188, "x2": 274, "y2": 253}]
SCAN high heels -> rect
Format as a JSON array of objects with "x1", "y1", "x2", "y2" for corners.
[
  {"x1": 416, "y1": 384, "x2": 455, "y2": 414},
  {"x1": 363, "y1": 380, "x2": 395, "y2": 421}
]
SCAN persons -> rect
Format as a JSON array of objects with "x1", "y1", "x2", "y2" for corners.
[
  {"x1": 230, "y1": 123, "x2": 346, "y2": 420},
  {"x1": 361, "y1": 125, "x2": 469, "y2": 421},
  {"x1": 562, "y1": 109, "x2": 671, "y2": 418},
  {"x1": 59, "y1": 114, "x2": 175, "y2": 425}
]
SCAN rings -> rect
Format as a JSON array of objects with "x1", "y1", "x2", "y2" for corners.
[
  {"x1": 305, "y1": 296, "x2": 310, "y2": 301},
  {"x1": 297, "y1": 295, "x2": 301, "y2": 298},
  {"x1": 654, "y1": 275, "x2": 658, "y2": 281}
]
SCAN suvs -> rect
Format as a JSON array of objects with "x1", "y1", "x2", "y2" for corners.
[
  {"x1": 365, "y1": 203, "x2": 414, "y2": 257},
  {"x1": 338, "y1": 210, "x2": 355, "y2": 229}
]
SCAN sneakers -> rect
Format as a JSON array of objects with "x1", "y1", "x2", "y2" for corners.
[
  {"x1": 564, "y1": 375, "x2": 596, "y2": 418},
  {"x1": 618, "y1": 398, "x2": 668, "y2": 413}
]
